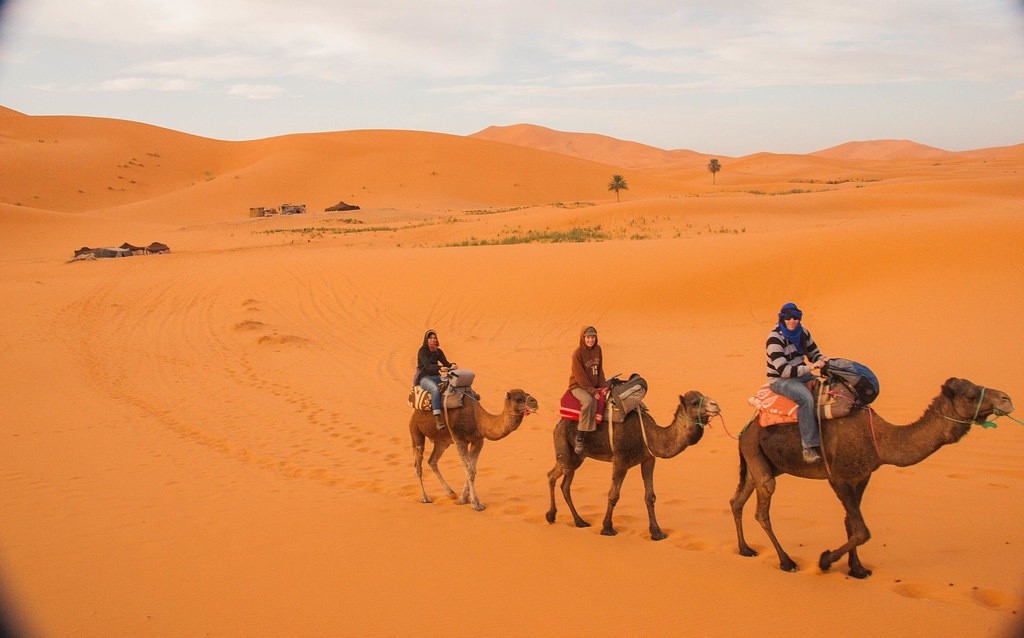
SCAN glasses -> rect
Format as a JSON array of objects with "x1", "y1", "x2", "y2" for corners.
[{"x1": 784, "y1": 315, "x2": 798, "y2": 320}]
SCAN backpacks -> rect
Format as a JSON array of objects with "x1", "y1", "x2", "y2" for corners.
[{"x1": 820, "y1": 357, "x2": 880, "y2": 405}]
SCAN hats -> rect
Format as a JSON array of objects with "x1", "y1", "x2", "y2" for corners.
[
  {"x1": 778, "y1": 303, "x2": 803, "y2": 320},
  {"x1": 583, "y1": 327, "x2": 597, "y2": 336}
]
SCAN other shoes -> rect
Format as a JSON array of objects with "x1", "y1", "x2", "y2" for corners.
[{"x1": 435, "y1": 414, "x2": 445, "y2": 431}]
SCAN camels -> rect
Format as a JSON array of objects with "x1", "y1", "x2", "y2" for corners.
[
  {"x1": 546, "y1": 389, "x2": 722, "y2": 543},
  {"x1": 409, "y1": 385, "x2": 540, "y2": 513},
  {"x1": 728, "y1": 375, "x2": 1017, "y2": 580}
]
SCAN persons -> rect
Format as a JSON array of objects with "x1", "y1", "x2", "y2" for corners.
[
  {"x1": 764, "y1": 303, "x2": 831, "y2": 463},
  {"x1": 568, "y1": 325, "x2": 607, "y2": 454},
  {"x1": 414, "y1": 329, "x2": 457, "y2": 430}
]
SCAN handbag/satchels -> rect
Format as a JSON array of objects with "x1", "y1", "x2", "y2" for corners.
[
  {"x1": 413, "y1": 371, "x2": 422, "y2": 386},
  {"x1": 446, "y1": 369, "x2": 475, "y2": 387},
  {"x1": 603, "y1": 373, "x2": 648, "y2": 424}
]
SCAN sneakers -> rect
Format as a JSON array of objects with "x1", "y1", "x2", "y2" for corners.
[
  {"x1": 574, "y1": 435, "x2": 584, "y2": 455},
  {"x1": 802, "y1": 448, "x2": 822, "y2": 464}
]
